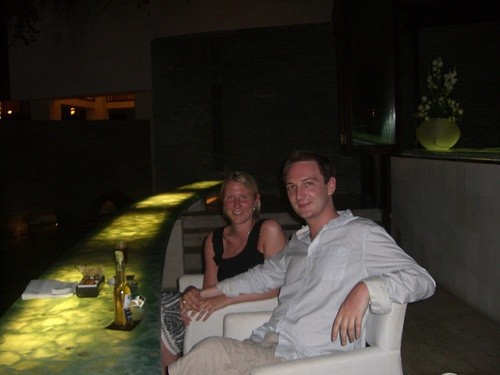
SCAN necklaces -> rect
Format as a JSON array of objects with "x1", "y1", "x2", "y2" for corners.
[{"x1": 229, "y1": 219, "x2": 254, "y2": 254}]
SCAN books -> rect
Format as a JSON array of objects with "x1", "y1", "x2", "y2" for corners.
[{"x1": 21, "y1": 279, "x2": 78, "y2": 301}]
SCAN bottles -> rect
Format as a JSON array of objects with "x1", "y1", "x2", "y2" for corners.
[
  {"x1": 114, "y1": 259, "x2": 133, "y2": 329},
  {"x1": 126, "y1": 275, "x2": 137, "y2": 299}
]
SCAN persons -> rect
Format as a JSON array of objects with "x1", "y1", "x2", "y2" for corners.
[
  {"x1": 159, "y1": 169, "x2": 288, "y2": 374},
  {"x1": 159, "y1": 146, "x2": 437, "y2": 374}
]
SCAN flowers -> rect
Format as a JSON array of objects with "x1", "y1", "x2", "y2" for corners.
[{"x1": 417, "y1": 55, "x2": 463, "y2": 121}]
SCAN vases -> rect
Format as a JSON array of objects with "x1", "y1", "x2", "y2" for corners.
[{"x1": 416, "y1": 119, "x2": 461, "y2": 153}]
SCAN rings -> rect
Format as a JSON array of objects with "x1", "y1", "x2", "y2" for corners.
[{"x1": 182, "y1": 300, "x2": 186, "y2": 303}]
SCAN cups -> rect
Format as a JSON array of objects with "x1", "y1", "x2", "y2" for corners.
[{"x1": 112, "y1": 240, "x2": 128, "y2": 265}]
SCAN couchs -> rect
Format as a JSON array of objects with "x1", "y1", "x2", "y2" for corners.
[
  {"x1": 178, "y1": 274, "x2": 280, "y2": 358},
  {"x1": 221, "y1": 302, "x2": 407, "y2": 375}
]
